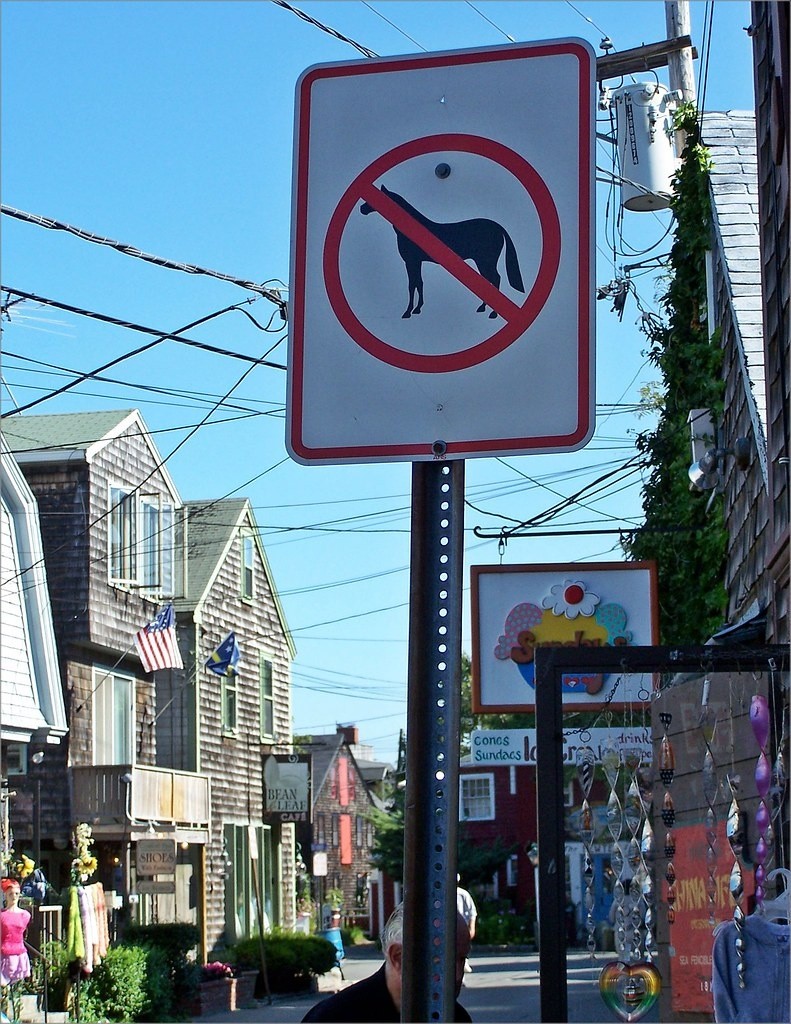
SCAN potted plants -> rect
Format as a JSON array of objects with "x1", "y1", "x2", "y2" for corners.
[{"x1": 325, "y1": 888, "x2": 345, "y2": 927}]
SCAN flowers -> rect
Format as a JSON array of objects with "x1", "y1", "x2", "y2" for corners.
[
  {"x1": 72, "y1": 822, "x2": 98, "y2": 877},
  {"x1": 11, "y1": 854, "x2": 35, "y2": 879}
]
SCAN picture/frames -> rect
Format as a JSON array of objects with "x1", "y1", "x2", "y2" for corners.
[{"x1": 470, "y1": 561, "x2": 663, "y2": 713}]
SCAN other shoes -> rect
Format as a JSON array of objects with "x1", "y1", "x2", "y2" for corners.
[{"x1": 464, "y1": 965, "x2": 473, "y2": 972}]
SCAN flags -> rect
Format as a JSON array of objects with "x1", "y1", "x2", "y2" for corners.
[
  {"x1": 132, "y1": 597, "x2": 184, "y2": 672},
  {"x1": 203, "y1": 630, "x2": 241, "y2": 677}
]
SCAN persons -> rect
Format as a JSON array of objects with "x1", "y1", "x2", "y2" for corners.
[
  {"x1": 300, "y1": 887, "x2": 478, "y2": 1024},
  {"x1": 0, "y1": 879, "x2": 31, "y2": 988}
]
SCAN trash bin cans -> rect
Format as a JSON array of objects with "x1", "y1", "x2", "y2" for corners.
[{"x1": 319, "y1": 927, "x2": 345, "y2": 959}]
